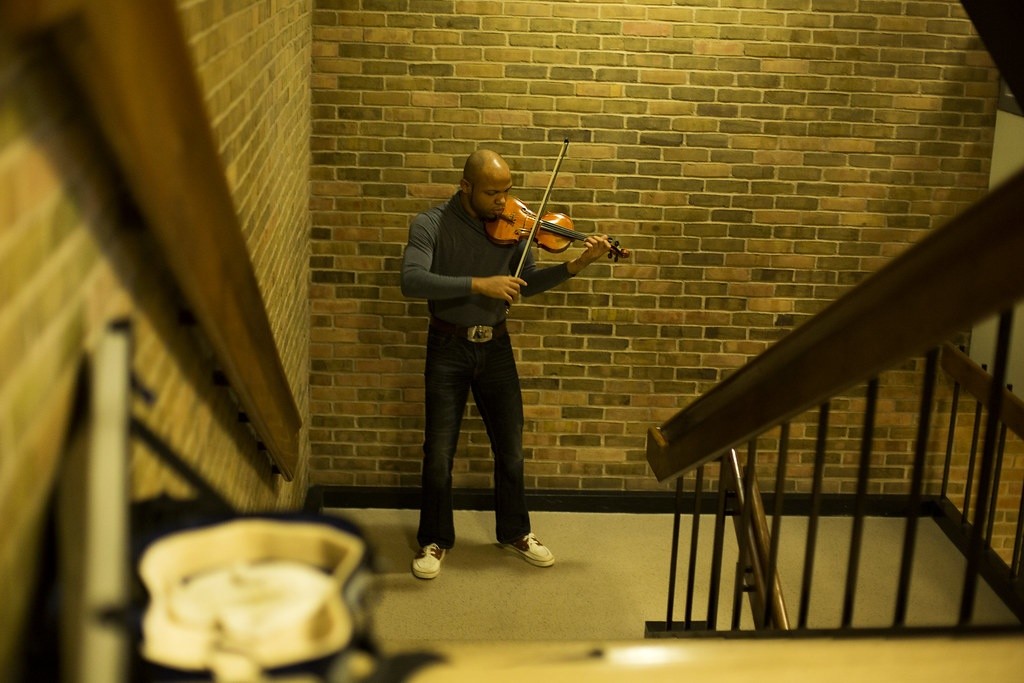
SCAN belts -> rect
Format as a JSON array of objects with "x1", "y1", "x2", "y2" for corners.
[{"x1": 430, "y1": 317, "x2": 506, "y2": 343}]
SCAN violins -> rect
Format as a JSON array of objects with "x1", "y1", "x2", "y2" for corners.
[{"x1": 484, "y1": 195, "x2": 630, "y2": 263}]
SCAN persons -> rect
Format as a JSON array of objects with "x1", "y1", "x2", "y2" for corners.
[{"x1": 401, "y1": 149, "x2": 612, "y2": 578}]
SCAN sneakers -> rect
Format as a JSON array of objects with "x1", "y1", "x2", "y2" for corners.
[
  {"x1": 411, "y1": 543, "x2": 446, "y2": 579},
  {"x1": 501, "y1": 533, "x2": 555, "y2": 567}
]
